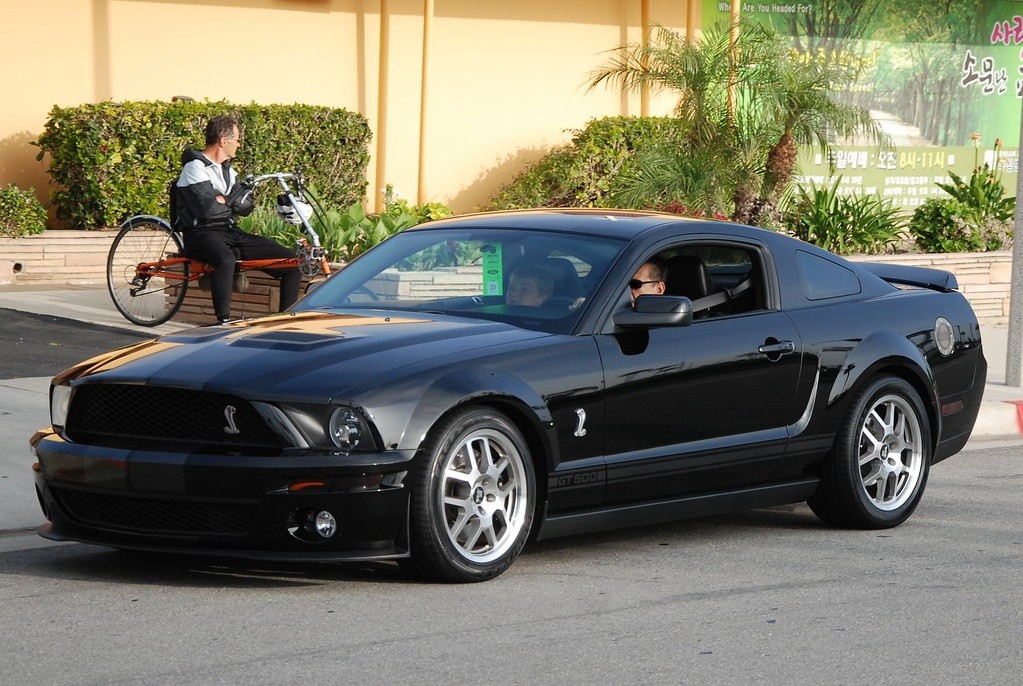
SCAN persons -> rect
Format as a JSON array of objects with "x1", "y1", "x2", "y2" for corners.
[
  {"x1": 175, "y1": 114, "x2": 304, "y2": 325},
  {"x1": 506, "y1": 264, "x2": 554, "y2": 307},
  {"x1": 570, "y1": 261, "x2": 665, "y2": 312}
]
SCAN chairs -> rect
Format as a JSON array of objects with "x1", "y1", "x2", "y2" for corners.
[
  {"x1": 546, "y1": 257, "x2": 581, "y2": 309},
  {"x1": 665, "y1": 256, "x2": 717, "y2": 320}
]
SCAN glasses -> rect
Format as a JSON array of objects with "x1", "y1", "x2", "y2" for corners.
[
  {"x1": 228, "y1": 137, "x2": 240, "y2": 143},
  {"x1": 630, "y1": 278, "x2": 660, "y2": 288}
]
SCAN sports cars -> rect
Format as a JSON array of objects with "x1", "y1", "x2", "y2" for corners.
[{"x1": 23, "y1": 211, "x2": 989, "y2": 582}]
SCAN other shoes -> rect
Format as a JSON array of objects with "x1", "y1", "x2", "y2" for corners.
[{"x1": 219, "y1": 318, "x2": 229, "y2": 323}]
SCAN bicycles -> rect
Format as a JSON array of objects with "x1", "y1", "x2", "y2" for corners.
[{"x1": 106, "y1": 170, "x2": 382, "y2": 329}]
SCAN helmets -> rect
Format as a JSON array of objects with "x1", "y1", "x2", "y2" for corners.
[{"x1": 277, "y1": 201, "x2": 313, "y2": 226}]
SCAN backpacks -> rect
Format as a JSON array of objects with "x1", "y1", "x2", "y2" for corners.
[{"x1": 170, "y1": 178, "x2": 178, "y2": 231}]
